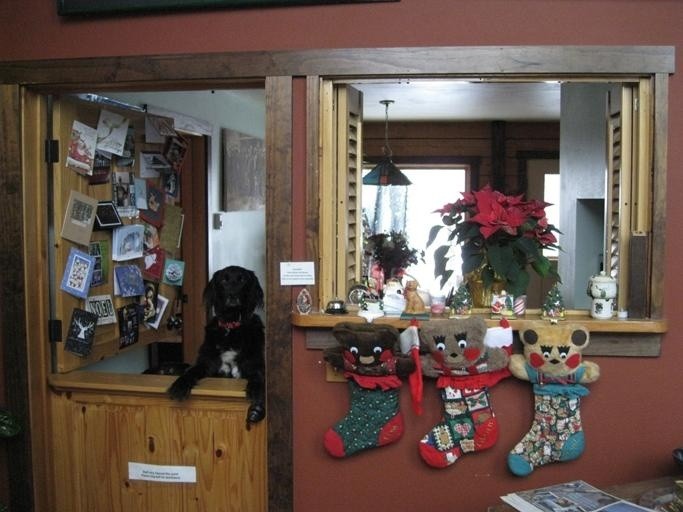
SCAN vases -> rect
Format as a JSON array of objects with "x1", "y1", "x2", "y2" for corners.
[{"x1": 464, "y1": 278, "x2": 509, "y2": 308}]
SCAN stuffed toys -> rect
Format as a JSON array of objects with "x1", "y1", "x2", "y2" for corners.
[
  {"x1": 320, "y1": 322, "x2": 413, "y2": 458},
  {"x1": 419, "y1": 316, "x2": 516, "y2": 469},
  {"x1": 506, "y1": 320, "x2": 599, "y2": 475}
]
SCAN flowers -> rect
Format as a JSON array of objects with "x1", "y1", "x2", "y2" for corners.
[{"x1": 424, "y1": 181, "x2": 568, "y2": 302}]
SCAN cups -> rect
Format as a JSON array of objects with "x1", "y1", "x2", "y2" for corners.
[{"x1": 587, "y1": 270, "x2": 617, "y2": 319}]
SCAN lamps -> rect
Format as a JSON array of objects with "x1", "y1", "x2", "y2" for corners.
[{"x1": 362, "y1": 100, "x2": 414, "y2": 186}]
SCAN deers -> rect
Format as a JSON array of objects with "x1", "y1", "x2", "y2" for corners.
[{"x1": 74, "y1": 317, "x2": 94, "y2": 339}]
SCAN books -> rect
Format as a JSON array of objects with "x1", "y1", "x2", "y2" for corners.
[
  {"x1": 501, "y1": 478, "x2": 654, "y2": 512},
  {"x1": 62, "y1": 111, "x2": 187, "y2": 356}
]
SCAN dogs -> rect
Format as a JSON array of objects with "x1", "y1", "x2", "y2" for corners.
[{"x1": 167, "y1": 266, "x2": 265, "y2": 407}]
